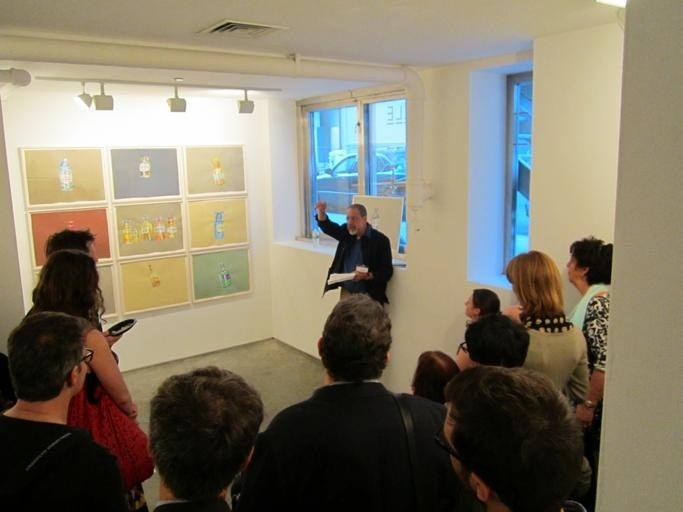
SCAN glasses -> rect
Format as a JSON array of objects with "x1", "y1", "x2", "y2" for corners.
[
  {"x1": 456, "y1": 341, "x2": 469, "y2": 356},
  {"x1": 432, "y1": 423, "x2": 474, "y2": 473},
  {"x1": 78, "y1": 347, "x2": 94, "y2": 364}
]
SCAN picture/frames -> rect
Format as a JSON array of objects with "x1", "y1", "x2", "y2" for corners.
[
  {"x1": 348, "y1": 194, "x2": 406, "y2": 260},
  {"x1": 19, "y1": 142, "x2": 255, "y2": 320}
]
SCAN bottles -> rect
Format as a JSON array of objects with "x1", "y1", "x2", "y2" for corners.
[{"x1": 55, "y1": 152, "x2": 235, "y2": 292}]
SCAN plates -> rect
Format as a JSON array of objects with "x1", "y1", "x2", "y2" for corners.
[{"x1": 107, "y1": 318, "x2": 137, "y2": 338}]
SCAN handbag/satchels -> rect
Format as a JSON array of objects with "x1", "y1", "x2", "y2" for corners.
[{"x1": 66, "y1": 379, "x2": 156, "y2": 495}]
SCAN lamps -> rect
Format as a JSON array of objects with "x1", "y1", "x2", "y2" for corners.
[{"x1": 67, "y1": 81, "x2": 256, "y2": 116}]
